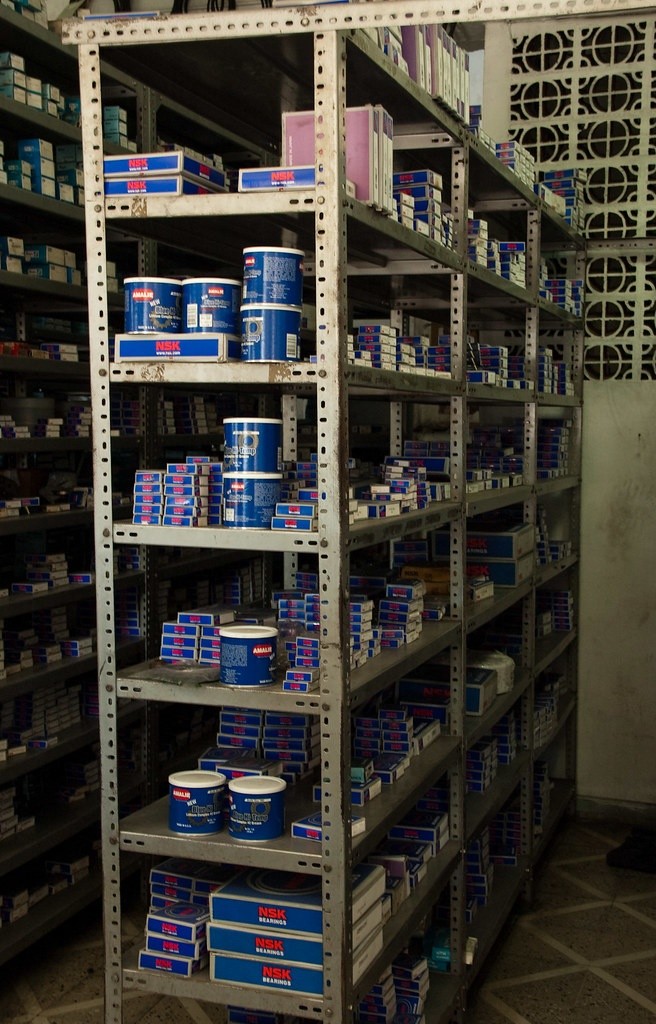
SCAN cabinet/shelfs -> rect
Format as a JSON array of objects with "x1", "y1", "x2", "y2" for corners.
[{"x1": 0, "y1": 0, "x2": 656, "y2": 1024}]
